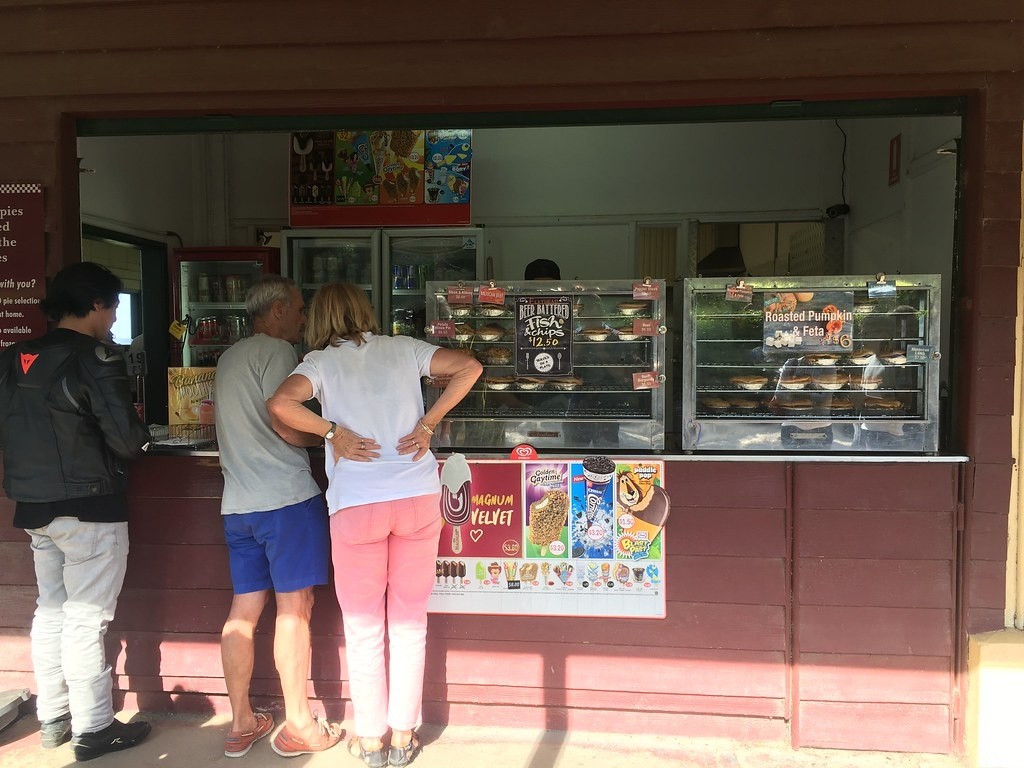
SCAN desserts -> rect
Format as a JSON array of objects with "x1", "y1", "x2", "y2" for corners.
[
  {"x1": 702, "y1": 348, "x2": 911, "y2": 409},
  {"x1": 445, "y1": 302, "x2": 649, "y2": 390},
  {"x1": 823, "y1": 305, "x2": 842, "y2": 334}
]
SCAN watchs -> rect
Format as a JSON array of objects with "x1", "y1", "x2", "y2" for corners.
[{"x1": 324, "y1": 421, "x2": 335, "y2": 439}]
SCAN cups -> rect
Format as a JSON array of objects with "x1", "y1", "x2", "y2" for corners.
[{"x1": 427, "y1": 187, "x2": 440, "y2": 203}]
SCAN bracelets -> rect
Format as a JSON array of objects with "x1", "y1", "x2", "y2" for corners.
[{"x1": 419, "y1": 418, "x2": 435, "y2": 434}]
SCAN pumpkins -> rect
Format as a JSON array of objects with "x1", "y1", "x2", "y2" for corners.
[{"x1": 766, "y1": 292, "x2": 813, "y2": 316}]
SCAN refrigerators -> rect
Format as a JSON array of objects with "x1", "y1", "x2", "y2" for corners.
[
  {"x1": 280, "y1": 228, "x2": 487, "y2": 414},
  {"x1": 166, "y1": 242, "x2": 278, "y2": 366}
]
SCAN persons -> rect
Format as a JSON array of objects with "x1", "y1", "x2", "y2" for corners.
[
  {"x1": 563, "y1": 295, "x2": 631, "y2": 448},
  {"x1": 499, "y1": 259, "x2": 571, "y2": 447},
  {"x1": 0, "y1": 263, "x2": 153, "y2": 761},
  {"x1": 215, "y1": 274, "x2": 341, "y2": 758},
  {"x1": 743, "y1": 305, "x2": 924, "y2": 448},
  {"x1": 268, "y1": 282, "x2": 483, "y2": 768}
]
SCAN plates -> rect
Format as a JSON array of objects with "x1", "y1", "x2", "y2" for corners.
[
  {"x1": 853, "y1": 306, "x2": 876, "y2": 313},
  {"x1": 616, "y1": 307, "x2": 640, "y2": 316},
  {"x1": 550, "y1": 382, "x2": 577, "y2": 391},
  {"x1": 450, "y1": 309, "x2": 471, "y2": 317},
  {"x1": 477, "y1": 334, "x2": 498, "y2": 340},
  {"x1": 586, "y1": 334, "x2": 609, "y2": 341},
  {"x1": 618, "y1": 335, "x2": 640, "y2": 341},
  {"x1": 857, "y1": 383, "x2": 879, "y2": 390},
  {"x1": 481, "y1": 308, "x2": 504, "y2": 317},
  {"x1": 487, "y1": 383, "x2": 509, "y2": 391},
  {"x1": 780, "y1": 384, "x2": 805, "y2": 390},
  {"x1": 818, "y1": 383, "x2": 845, "y2": 390},
  {"x1": 514, "y1": 381, "x2": 539, "y2": 390},
  {"x1": 884, "y1": 358, "x2": 909, "y2": 364},
  {"x1": 848, "y1": 358, "x2": 869, "y2": 365},
  {"x1": 450, "y1": 334, "x2": 473, "y2": 340},
  {"x1": 738, "y1": 383, "x2": 763, "y2": 390},
  {"x1": 807, "y1": 358, "x2": 839, "y2": 366}
]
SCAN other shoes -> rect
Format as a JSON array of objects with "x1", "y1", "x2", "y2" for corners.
[
  {"x1": 348, "y1": 736, "x2": 388, "y2": 768},
  {"x1": 389, "y1": 732, "x2": 420, "y2": 767}
]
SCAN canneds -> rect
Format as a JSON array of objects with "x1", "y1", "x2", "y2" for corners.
[
  {"x1": 196, "y1": 271, "x2": 253, "y2": 344},
  {"x1": 390, "y1": 264, "x2": 431, "y2": 290},
  {"x1": 312, "y1": 254, "x2": 371, "y2": 284},
  {"x1": 134, "y1": 402, "x2": 144, "y2": 422}
]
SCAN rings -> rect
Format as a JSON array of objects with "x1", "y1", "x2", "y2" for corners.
[
  {"x1": 416, "y1": 444, "x2": 419, "y2": 448},
  {"x1": 362, "y1": 438, "x2": 364, "y2": 442},
  {"x1": 362, "y1": 444, "x2": 365, "y2": 449},
  {"x1": 412, "y1": 440, "x2": 415, "y2": 444}
]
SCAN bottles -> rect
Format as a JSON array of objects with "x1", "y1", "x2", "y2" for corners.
[{"x1": 194, "y1": 348, "x2": 222, "y2": 366}]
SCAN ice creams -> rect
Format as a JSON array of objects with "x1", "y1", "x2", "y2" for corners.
[{"x1": 583, "y1": 455, "x2": 616, "y2": 528}]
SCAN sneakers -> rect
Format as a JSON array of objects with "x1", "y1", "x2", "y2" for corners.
[
  {"x1": 224, "y1": 712, "x2": 274, "y2": 757},
  {"x1": 72, "y1": 717, "x2": 151, "y2": 761},
  {"x1": 38, "y1": 712, "x2": 72, "y2": 748},
  {"x1": 270, "y1": 709, "x2": 341, "y2": 757}
]
